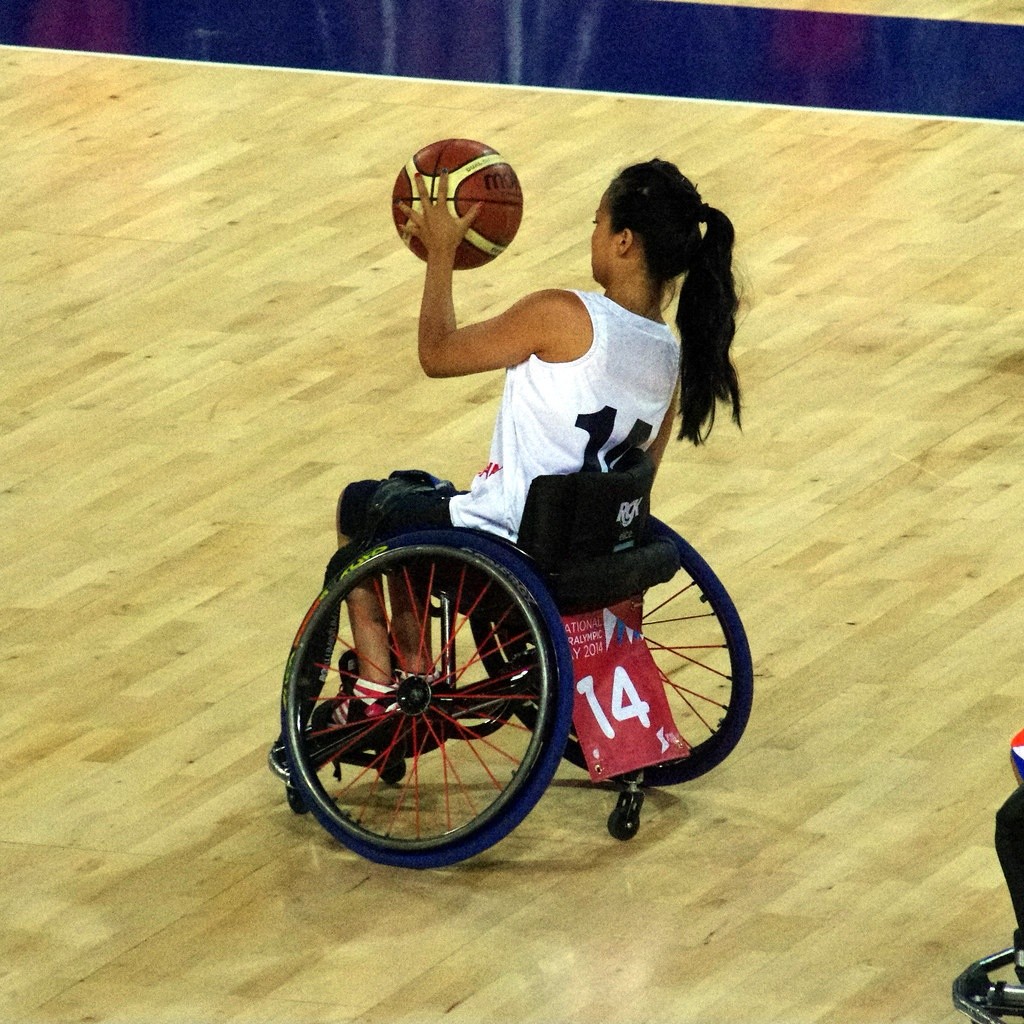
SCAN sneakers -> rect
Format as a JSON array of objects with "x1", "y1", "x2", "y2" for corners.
[
  {"x1": 314, "y1": 650, "x2": 404, "y2": 733},
  {"x1": 388, "y1": 636, "x2": 442, "y2": 695}
]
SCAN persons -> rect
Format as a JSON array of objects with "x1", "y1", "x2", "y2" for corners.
[
  {"x1": 994, "y1": 728, "x2": 1024, "y2": 984},
  {"x1": 308, "y1": 157, "x2": 745, "y2": 730}
]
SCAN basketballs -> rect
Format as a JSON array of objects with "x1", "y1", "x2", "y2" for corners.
[{"x1": 389, "y1": 135, "x2": 525, "y2": 273}]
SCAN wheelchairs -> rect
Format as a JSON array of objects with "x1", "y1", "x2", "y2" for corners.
[{"x1": 265, "y1": 446, "x2": 754, "y2": 868}]
